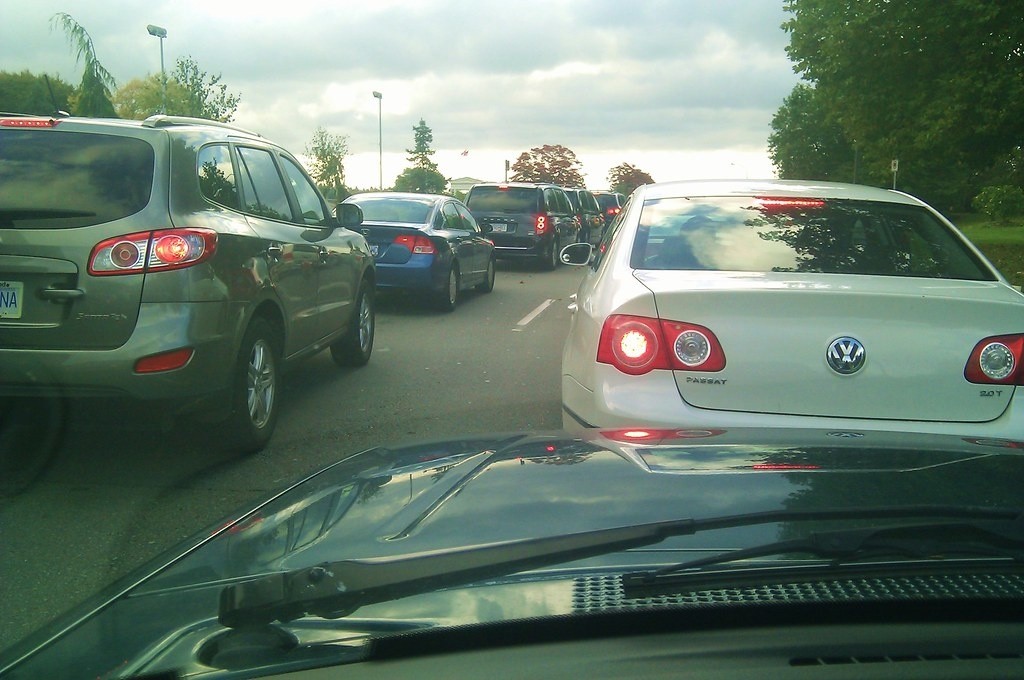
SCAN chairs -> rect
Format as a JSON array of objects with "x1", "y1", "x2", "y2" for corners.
[
  {"x1": 839, "y1": 245, "x2": 910, "y2": 276},
  {"x1": 660, "y1": 236, "x2": 717, "y2": 269}
]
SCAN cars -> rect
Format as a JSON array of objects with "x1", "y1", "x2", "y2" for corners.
[
  {"x1": 558, "y1": 178, "x2": 1024, "y2": 450},
  {"x1": 328, "y1": 193, "x2": 499, "y2": 313}
]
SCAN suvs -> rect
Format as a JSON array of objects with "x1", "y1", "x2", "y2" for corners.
[
  {"x1": 459, "y1": 183, "x2": 583, "y2": 269},
  {"x1": 538, "y1": 179, "x2": 630, "y2": 259},
  {"x1": 0, "y1": 115, "x2": 377, "y2": 453}
]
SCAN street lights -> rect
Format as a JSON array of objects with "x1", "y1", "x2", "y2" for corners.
[
  {"x1": 146, "y1": 24, "x2": 169, "y2": 117},
  {"x1": 373, "y1": 90, "x2": 386, "y2": 192}
]
